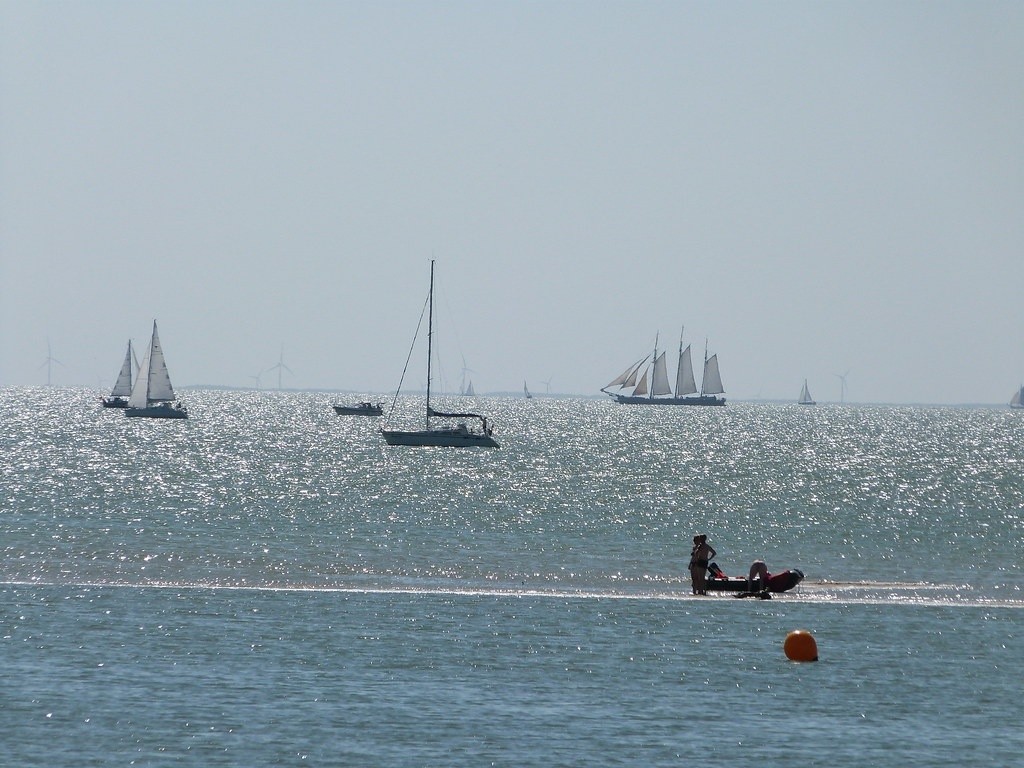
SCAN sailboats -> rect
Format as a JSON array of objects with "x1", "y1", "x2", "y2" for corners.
[
  {"x1": 600, "y1": 325, "x2": 726, "y2": 406},
  {"x1": 1006, "y1": 385, "x2": 1024, "y2": 409},
  {"x1": 460, "y1": 380, "x2": 475, "y2": 396},
  {"x1": 103, "y1": 339, "x2": 152, "y2": 407},
  {"x1": 380, "y1": 260, "x2": 501, "y2": 447},
  {"x1": 125, "y1": 319, "x2": 187, "y2": 419},
  {"x1": 797, "y1": 378, "x2": 817, "y2": 405}
]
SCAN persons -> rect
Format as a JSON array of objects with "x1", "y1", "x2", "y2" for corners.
[
  {"x1": 695, "y1": 534, "x2": 716, "y2": 594},
  {"x1": 748, "y1": 561, "x2": 768, "y2": 593},
  {"x1": 480, "y1": 417, "x2": 487, "y2": 435},
  {"x1": 688, "y1": 536, "x2": 699, "y2": 595}
]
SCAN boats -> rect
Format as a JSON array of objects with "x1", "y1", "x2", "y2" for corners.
[
  {"x1": 692, "y1": 569, "x2": 805, "y2": 593},
  {"x1": 332, "y1": 402, "x2": 385, "y2": 416}
]
